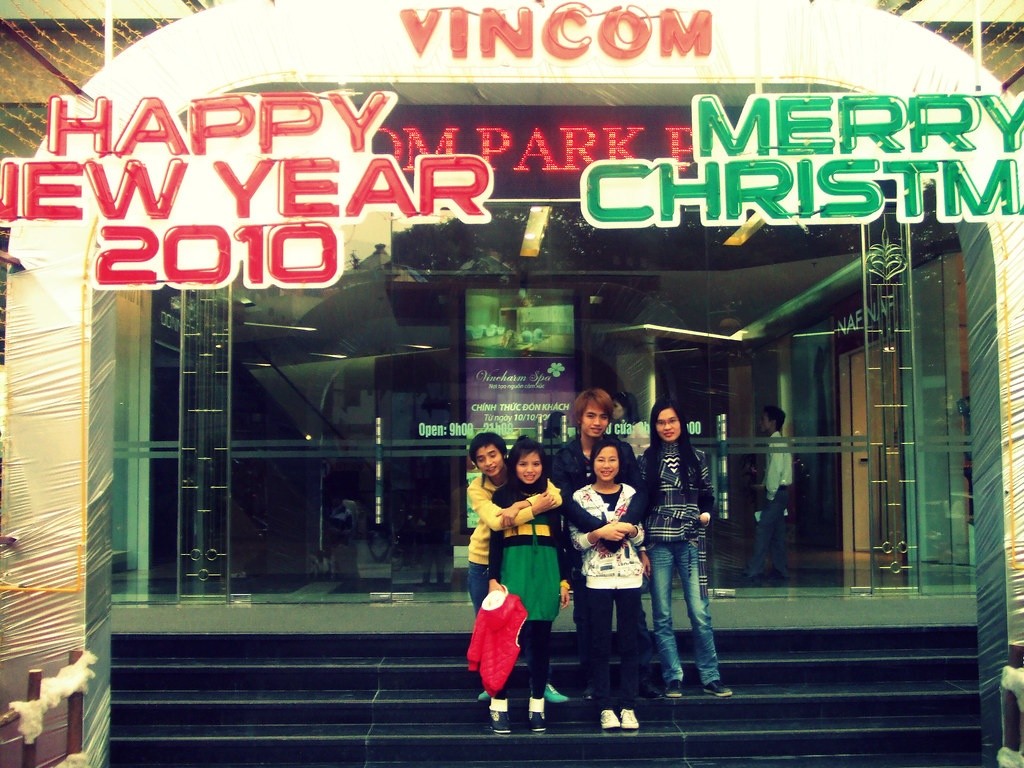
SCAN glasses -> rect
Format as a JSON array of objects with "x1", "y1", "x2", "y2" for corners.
[{"x1": 656, "y1": 419, "x2": 679, "y2": 425}]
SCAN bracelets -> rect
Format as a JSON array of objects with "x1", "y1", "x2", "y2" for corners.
[{"x1": 559, "y1": 581, "x2": 570, "y2": 589}]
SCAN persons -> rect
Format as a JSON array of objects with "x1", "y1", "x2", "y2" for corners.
[
  {"x1": 566, "y1": 438, "x2": 648, "y2": 729},
  {"x1": 550, "y1": 387, "x2": 665, "y2": 700},
  {"x1": 533, "y1": 410, "x2": 574, "y2": 457},
  {"x1": 465, "y1": 430, "x2": 570, "y2": 704},
  {"x1": 638, "y1": 394, "x2": 735, "y2": 698},
  {"x1": 736, "y1": 403, "x2": 795, "y2": 580},
  {"x1": 485, "y1": 434, "x2": 573, "y2": 733},
  {"x1": 307, "y1": 481, "x2": 456, "y2": 589},
  {"x1": 609, "y1": 392, "x2": 640, "y2": 444}
]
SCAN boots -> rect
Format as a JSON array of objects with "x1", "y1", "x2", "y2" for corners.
[
  {"x1": 489, "y1": 697, "x2": 511, "y2": 733},
  {"x1": 528, "y1": 697, "x2": 546, "y2": 731}
]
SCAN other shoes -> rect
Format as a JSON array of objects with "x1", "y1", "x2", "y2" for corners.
[{"x1": 478, "y1": 690, "x2": 491, "y2": 700}]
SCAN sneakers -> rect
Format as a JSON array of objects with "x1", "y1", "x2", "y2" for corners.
[
  {"x1": 601, "y1": 709, "x2": 621, "y2": 729},
  {"x1": 531, "y1": 683, "x2": 568, "y2": 703},
  {"x1": 704, "y1": 680, "x2": 732, "y2": 697},
  {"x1": 664, "y1": 679, "x2": 682, "y2": 697},
  {"x1": 620, "y1": 709, "x2": 639, "y2": 729}
]
